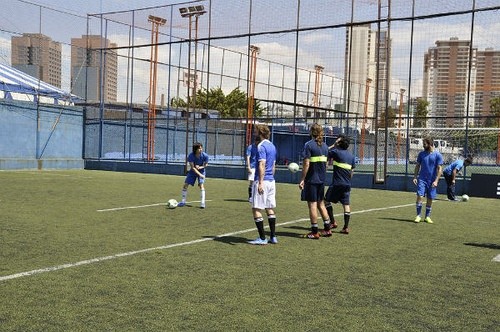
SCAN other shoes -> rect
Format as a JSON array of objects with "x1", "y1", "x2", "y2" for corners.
[
  {"x1": 248, "y1": 197, "x2": 252, "y2": 203},
  {"x1": 179, "y1": 202, "x2": 185, "y2": 206},
  {"x1": 449, "y1": 198, "x2": 460, "y2": 201},
  {"x1": 200, "y1": 203, "x2": 205, "y2": 207}
]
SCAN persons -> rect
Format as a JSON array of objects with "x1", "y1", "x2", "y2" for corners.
[
  {"x1": 412, "y1": 137, "x2": 443, "y2": 223},
  {"x1": 247, "y1": 123, "x2": 278, "y2": 245},
  {"x1": 442, "y1": 156, "x2": 473, "y2": 201},
  {"x1": 178, "y1": 141, "x2": 208, "y2": 208},
  {"x1": 246, "y1": 137, "x2": 261, "y2": 203},
  {"x1": 324, "y1": 134, "x2": 357, "y2": 234},
  {"x1": 299, "y1": 122, "x2": 335, "y2": 240}
]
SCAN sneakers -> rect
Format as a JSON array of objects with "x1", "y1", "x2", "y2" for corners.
[
  {"x1": 268, "y1": 237, "x2": 278, "y2": 244},
  {"x1": 415, "y1": 216, "x2": 422, "y2": 222},
  {"x1": 306, "y1": 233, "x2": 319, "y2": 239},
  {"x1": 329, "y1": 221, "x2": 338, "y2": 229},
  {"x1": 424, "y1": 217, "x2": 433, "y2": 224},
  {"x1": 249, "y1": 237, "x2": 267, "y2": 245},
  {"x1": 340, "y1": 228, "x2": 349, "y2": 234},
  {"x1": 319, "y1": 230, "x2": 331, "y2": 237}
]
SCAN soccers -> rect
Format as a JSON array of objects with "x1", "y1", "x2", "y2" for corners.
[
  {"x1": 288, "y1": 162, "x2": 299, "y2": 173},
  {"x1": 167, "y1": 198, "x2": 178, "y2": 209},
  {"x1": 462, "y1": 194, "x2": 469, "y2": 201}
]
